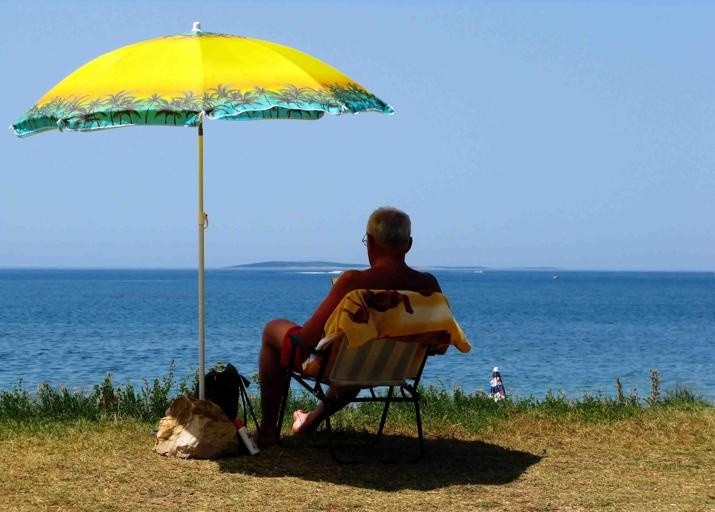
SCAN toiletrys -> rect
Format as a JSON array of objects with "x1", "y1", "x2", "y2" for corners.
[{"x1": 235, "y1": 417, "x2": 262, "y2": 457}]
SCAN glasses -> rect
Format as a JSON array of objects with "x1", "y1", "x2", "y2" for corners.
[{"x1": 362, "y1": 232, "x2": 372, "y2": 249}]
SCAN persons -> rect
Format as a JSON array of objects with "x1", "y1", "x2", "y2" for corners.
[{"x1": 250, "y1": 206, "x2": 442, "y2": 447}]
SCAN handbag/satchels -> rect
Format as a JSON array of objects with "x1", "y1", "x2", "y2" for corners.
[{"x1": 194, "y1": 363, "x2": 242, "y2": 427}]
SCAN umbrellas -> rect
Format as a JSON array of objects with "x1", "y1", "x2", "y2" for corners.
[{"x1": 9, "y1": 20, "x2": 397, "y2": 401}]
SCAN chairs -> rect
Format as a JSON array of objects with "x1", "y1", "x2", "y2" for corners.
[{"x1": 274, "y1": 288, "x2": 474, "y2": 465}]
